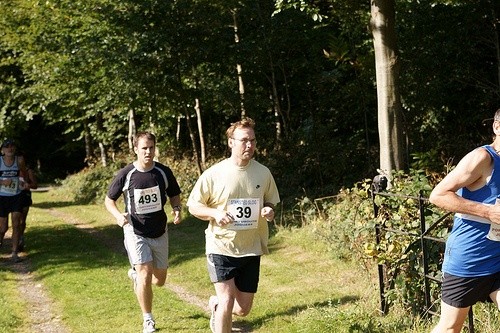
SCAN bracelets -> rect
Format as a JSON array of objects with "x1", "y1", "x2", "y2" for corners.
[
  {"x1": 175, "y1": 205, "x2": 181, "y2": 209},
  {"x1": 266, "y1": 205, "x2": 274, "y2": 211}
]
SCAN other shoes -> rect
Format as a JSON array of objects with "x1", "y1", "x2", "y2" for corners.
[{"x1": 12, "y1": 254, "x2": 19, "y2": 262}]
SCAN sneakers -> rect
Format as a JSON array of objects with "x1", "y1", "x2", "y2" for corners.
[
  {"x1": 142, "y1": 318, "x2": 155, "y2": 333},
  {"x1": 209, "y1": 295, "x2": 219, "y2": 333},
  {"x1": 128, "y1": 269, "x2": 137, "y2": 293}
]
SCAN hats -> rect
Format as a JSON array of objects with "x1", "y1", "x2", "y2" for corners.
[{"x1": 2, "y1": 140, "x2": 16, "y2": 148}]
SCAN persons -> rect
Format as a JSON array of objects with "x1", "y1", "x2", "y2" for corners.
[
  {"x1": 0, "y1": 141, "x2": 37, "y2": 263},
  {"x1": 105, "y1": 132, "x2": 182, "y2": 333},
  {"x1": 186, "y1": 118, "x2": 281, "y2": 333},
  {"x1": 429, "y1": 109, "x2": 500, "y2": 333}
]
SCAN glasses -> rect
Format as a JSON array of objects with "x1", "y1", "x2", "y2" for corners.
[{"x1": 232, "y1": 137, "x2": 257, "y2": 146}]
